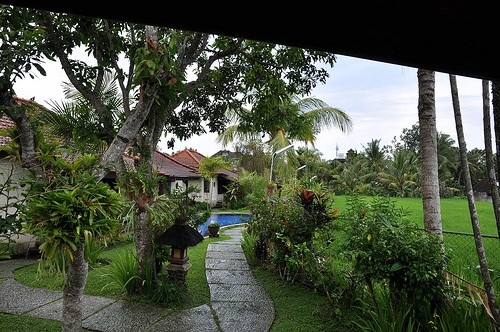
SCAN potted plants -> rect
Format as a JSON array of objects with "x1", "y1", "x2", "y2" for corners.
[{"x1": 208, "y1": 222, "x2": 220, "y2": 238}]
